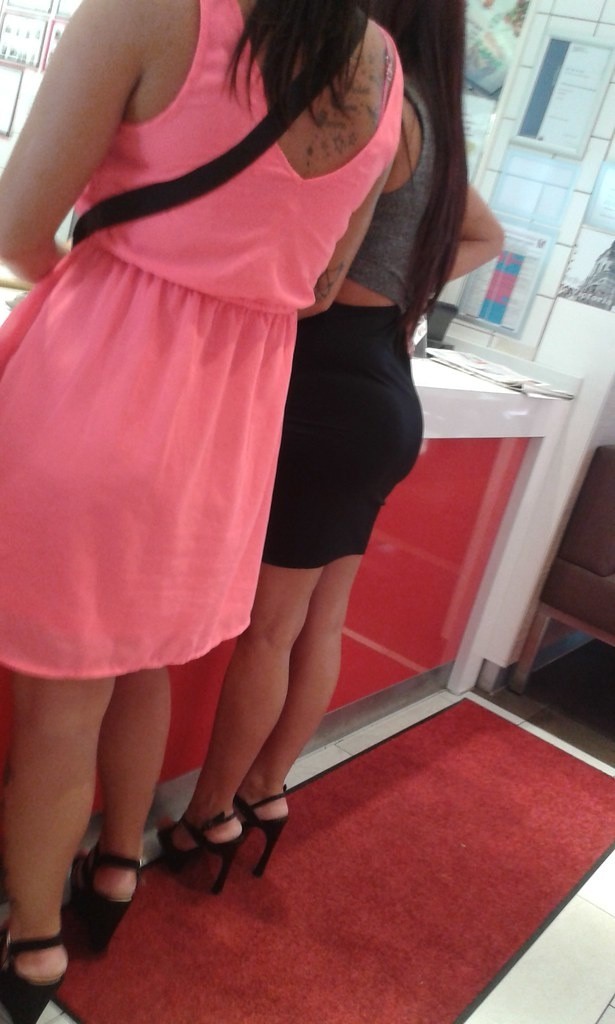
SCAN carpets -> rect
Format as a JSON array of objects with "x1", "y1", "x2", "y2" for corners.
[{"x1": 0, "y1": 696, "x2": 615, "y2": 1024}]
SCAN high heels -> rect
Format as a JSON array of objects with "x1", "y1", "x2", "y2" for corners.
[
  {"x1": 0, "y1": 928, "x2": 64, "y2": 1024},
  {"x1": 234, "y1": 785, "x2": 289, "y2": 878},
  {"x1": 157, "y1": 812, "x2": 246, "y2": 894},
  {"x1": 71, "y1": 841, "x2": 140, "y2": 952}
]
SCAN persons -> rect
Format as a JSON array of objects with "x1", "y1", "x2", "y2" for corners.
[
  {"x1": 161, "y1": 0, "x2": 506, "y2": 898},
  {"x1": 0, "y1": 0, "x2": 407, "y2": 1024}
]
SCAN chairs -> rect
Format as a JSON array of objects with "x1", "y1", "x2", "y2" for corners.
[{"x1": 505, "y1": 443, "x2": 615, "y2": 694}]
